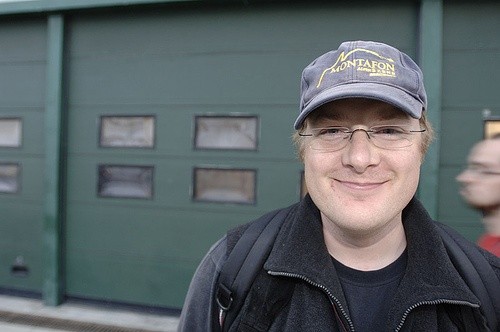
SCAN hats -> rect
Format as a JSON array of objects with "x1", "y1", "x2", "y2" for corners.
[{"x1": 293, "y1": 40, "x2": 427, "y2": 130}]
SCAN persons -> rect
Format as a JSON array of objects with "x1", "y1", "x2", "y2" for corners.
[
  {"x1": 456, "y1": 132, "x2": 500, "y2": 259},
  {"x1": 178, "y1": 40, "x2": 500, "y2": 332}
]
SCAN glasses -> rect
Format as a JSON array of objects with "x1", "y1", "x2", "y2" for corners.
[
  {"x1": 298, "y1": 116, "x2": 426, "y2": 151},
  {"x1": 464, "y1": 165, "x2": 500, "y2": 178}
]
